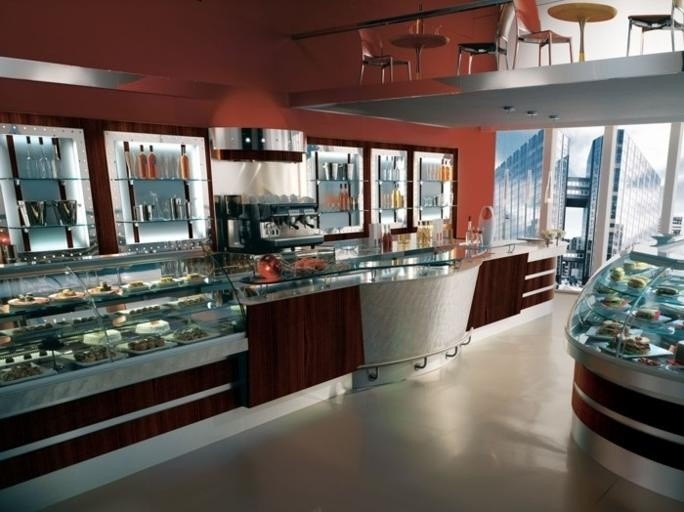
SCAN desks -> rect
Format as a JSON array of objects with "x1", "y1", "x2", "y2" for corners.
[
  {"x1": 391, "y1": 33, "x2": 450, "y2": 81},
  {"x1": 548, "y1": 3, "x2": 618, "y2": 62}
]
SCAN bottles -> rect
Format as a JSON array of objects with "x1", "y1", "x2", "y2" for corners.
[
  {"x1": 463, "y1": 216, "x2": 484, "y2": 247},
  {"x1": 417, "y1": 220, "x2": 446, "y2": 241},
  {"x1": 148, "y1": 190, "x2": 172, "y2": 222},
  {"x1": 384, "y1": 155, "x2": 401, "y2": 182},
  {"x1": 441, "y1": 159, "x2": 451, "y2": 181},
  {"x1": 139, "y1": 143, "x2": 191, "y2": 180},
  {"x1": 384, "y1": 244, "x2": 393, "y2": 254},
  {"x1": 346, "y1": 153, "x2": 352, "y2": 164},
  {"x1": 380, "y1": 224, "x2": 394, "y2": 244},
  {"x1": 415, "y1": 2, "x2": 424, "y2": 34},
  {"x1": 339, "y1": 184, "x2": 351, "y2": 211},
  {"x1": 392, "y1": 184, "x2": 405, "y2": 209},
  {"x1": 26, "y1": 136, "x2": 64, "y2": 179}
]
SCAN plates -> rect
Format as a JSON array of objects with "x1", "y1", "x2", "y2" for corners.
[
  {"x1": 8, "y1": 271, "x2": 206, "y2": 309},
  {"x1": 584, "y1": 260, "x2": 684, "y2": 370}
]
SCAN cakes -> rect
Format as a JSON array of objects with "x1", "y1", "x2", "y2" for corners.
[{"x1": 598, "y1": 261, "x2": 678, "y2": 355}]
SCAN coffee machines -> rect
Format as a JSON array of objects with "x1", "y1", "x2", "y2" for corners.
[{"x1": 222, "y1": 192, "x2": 325, "y2": 249}]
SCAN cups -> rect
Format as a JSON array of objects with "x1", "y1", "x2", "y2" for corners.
[
  {"x1": 171, "y1": 197, "x2": 192, "y2": 221},
  {"x1": 18, "y1": 200, "x2": 45, "y2": 227},
  {"x1": 134, "y1": 204, "x2": 156, "y2": 222},
  {"x1": 52, "y1": 197, "x2": 78, "y2": 226},
  {"x1": 324, "y1": 162, "x2": 356, "y2": 180}
]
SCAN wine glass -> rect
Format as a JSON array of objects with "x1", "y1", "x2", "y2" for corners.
[
  {"x1": 426, "y1": 164, "x2": 440, "y2": 181},
  {"x1": 125, "y1": 152, "x2": 136, "y2": 181},
  {"x1": 423, "y1": 192, "x2": 455, "y2": 207}
]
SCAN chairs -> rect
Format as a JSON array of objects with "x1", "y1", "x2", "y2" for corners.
[
  {"x1": 357, "y1": 29, "x2": 412, "y2": 86},
  {"x1": 627, "y1": 1, "x2": 684, "y2": 57},
  {"x1": 457, "y1": 1, "x2": 515, "y2": 75},
  {"x1": 511, "y1": 1, "x2": 573, "y2": 69}
]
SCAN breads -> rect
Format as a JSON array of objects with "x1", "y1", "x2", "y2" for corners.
[{"x1": 0, "y1": 272, "x2": 207, "y2": 381}]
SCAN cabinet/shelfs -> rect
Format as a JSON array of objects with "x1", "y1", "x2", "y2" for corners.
[
  {"x1": 304, "y1": 135, "x2": 459, "y2": 242},
  {"x1": 0, "y1": 257, "x2": 243, "y2": 392},
  {"x1": 564, "y1": 250, "x2": 684, "y2": 379},
  {"x1": 0, "y1": 112, "x2": 217, "y2": 265}
]
